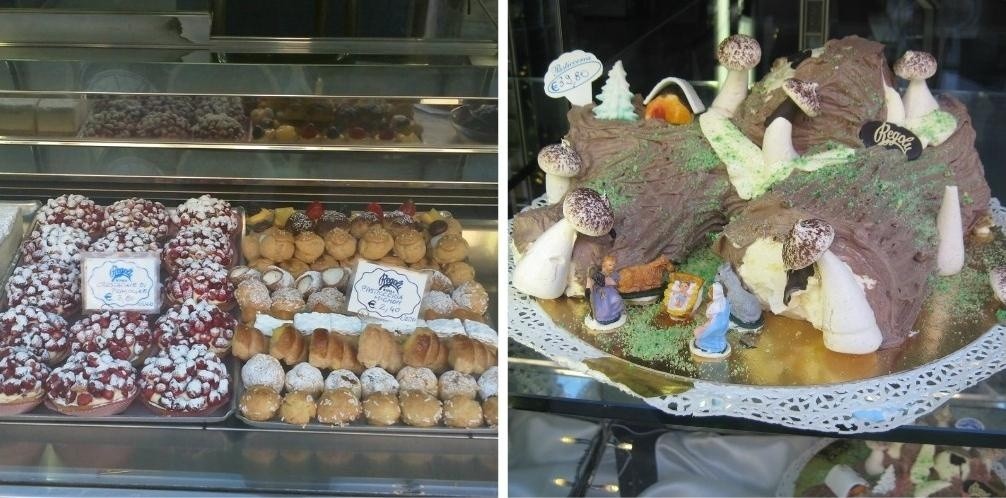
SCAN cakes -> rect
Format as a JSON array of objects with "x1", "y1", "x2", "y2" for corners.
[
  {"x1": 0, "y1": 194, "x2": 239, "y2": 418},
  {"x1": 80, "y1": 93, "x2": 246, "y2": 145},
  {"x1": 511, "y1": 28, "x2": 994, "y2": 353}
]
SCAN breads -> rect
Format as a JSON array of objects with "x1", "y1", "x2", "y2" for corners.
[
  {"x1": 234, "y1": 206, "x2": 500, "y2": 426},
  {"x1": 248, "y1": 96, "x2": 422, "y2": 147}
]
